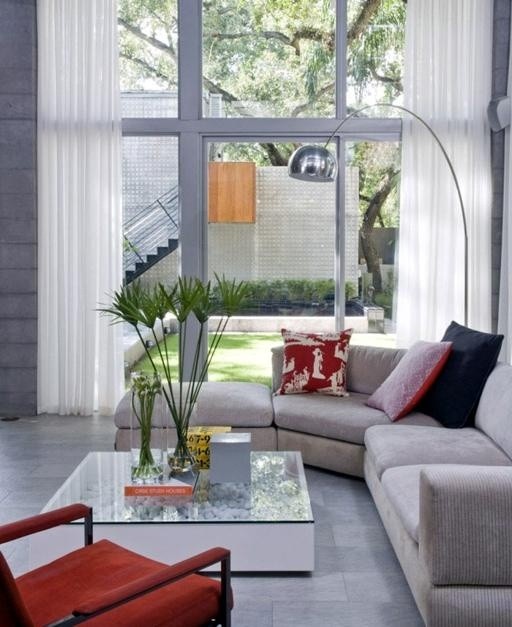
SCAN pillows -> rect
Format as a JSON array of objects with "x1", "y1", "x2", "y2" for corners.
[
  {"x1": 271, "y1": 326, "x2": 355, "y2": 398},
  {"x1": 363, "y1": 319, "x2": 505, "y2": 428}
]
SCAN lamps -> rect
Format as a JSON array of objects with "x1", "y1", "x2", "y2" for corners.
[{"x1": 285, "y1": 101, "x2": 469, "y2": 328}]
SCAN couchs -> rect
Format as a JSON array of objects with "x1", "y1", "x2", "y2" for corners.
[{"x1": 114, "y1": 343, "x2": 509, "y2": 626}]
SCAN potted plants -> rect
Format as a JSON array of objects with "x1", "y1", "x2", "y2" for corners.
[{"x1": 91, "y1": 269, "x2": 254, "y2": 485}]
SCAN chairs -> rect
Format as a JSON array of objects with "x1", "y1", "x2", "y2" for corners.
[{"x1": 2, "y1": 503, "x2": 235, "y2": 625}]
